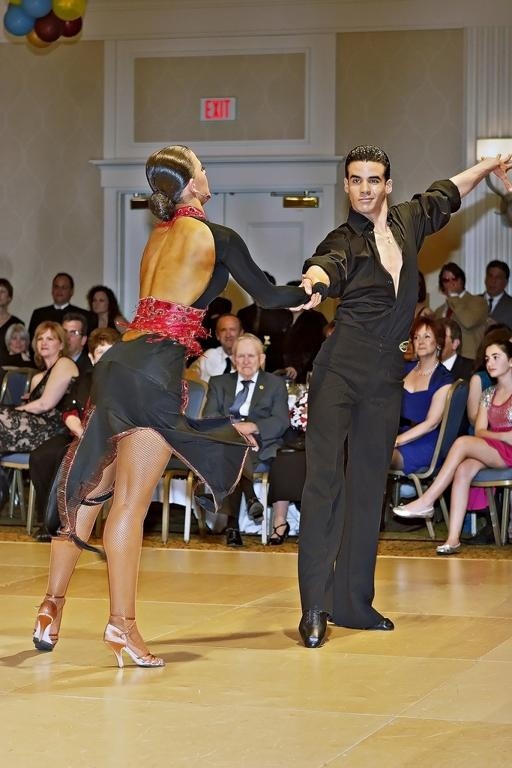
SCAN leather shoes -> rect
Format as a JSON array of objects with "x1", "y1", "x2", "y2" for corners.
[
  {"x1": 436, "y1": 542, "x2": 461, "y2": 554},
  {"x1": 247, "y1": 498, "x2": 264, "y2": 524},
  {"x1": 299, "y1": 610, "x2": 327, "y2": 647},
  {"x1": 372, "y1": 618, "x2": 394, "y2": 630},
  {"x1": 393, "y1": 505, "x2": 434, "y2": 518},
  {"x1": 226, "y1": 528, "x2": 243, "y2": 547}
]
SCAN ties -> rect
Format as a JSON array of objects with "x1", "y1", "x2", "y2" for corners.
[
  {"x1": 229, "y1": 381, "x2": 254, "y2": 419},
  {"x1": 488, "y1": 298, "x2": 493, "y2": 313},
  {"x1": 224, "y1": 357, "x2": 231, "y2": 373}
]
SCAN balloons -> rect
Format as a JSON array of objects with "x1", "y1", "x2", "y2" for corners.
[{"x1": 3, "y1": 0, "x2": 87, "y2": 56}]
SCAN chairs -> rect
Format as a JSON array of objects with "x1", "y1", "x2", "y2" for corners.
[{"x1": 0, "y1": 366, "x2": 512, "y2": 546}]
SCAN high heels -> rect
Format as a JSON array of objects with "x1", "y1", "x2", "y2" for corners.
[
  {"x1": 33, "y1": 600, "x2": 58, "y2": 651},
  {"x1": 104, "y1": 617, "x2": 165, "y2": 668},
  {"x1": 270, "y1": 522, "x2": 290, "y2": 545}
]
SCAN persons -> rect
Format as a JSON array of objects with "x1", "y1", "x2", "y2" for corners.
[
  {"x1": 287, "y1": 144, "x2": 512, "y2": 647},
  {"x1": 32, "y1": 145, "x2": 332, "y2": 668},
  {"x1": 0, "y1": 272, "x2": 126, "y2": 543},
  {"x1": 184, "y1": 271, "x2": 329, "y2": 548},
  {"x1": 389, "y1": 261, "x2": 512, "y2": 556}
]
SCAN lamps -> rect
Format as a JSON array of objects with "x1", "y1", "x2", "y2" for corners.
[{"x1": 471, "y1": 135, "x2": 510, "y2": 229}]
